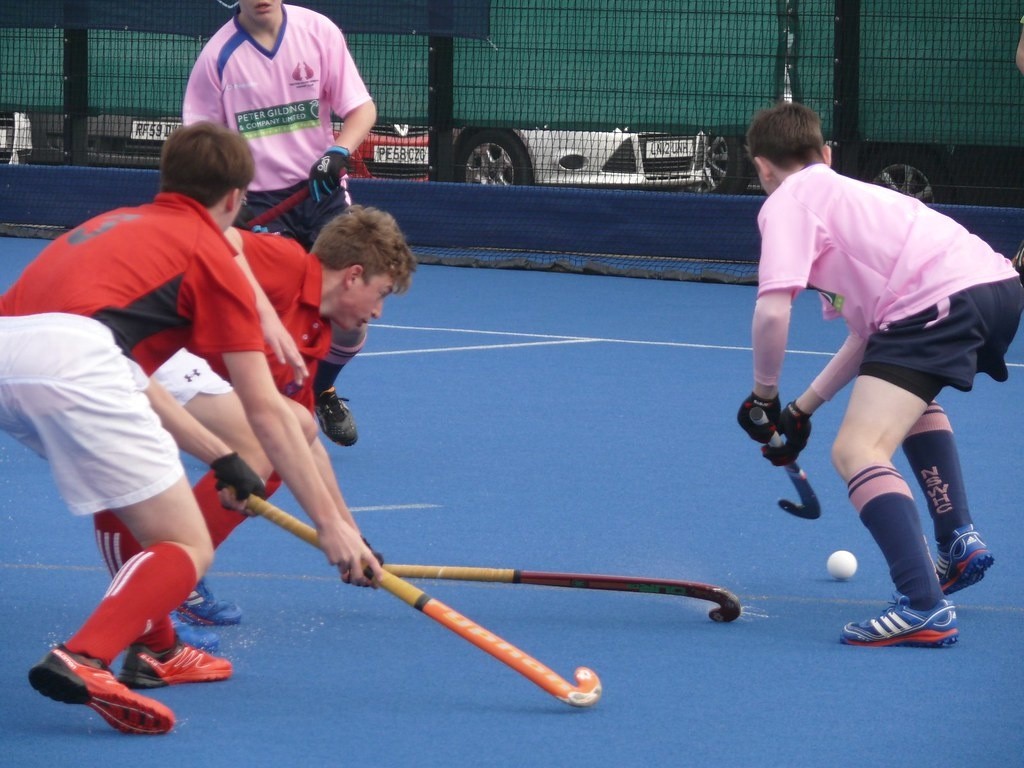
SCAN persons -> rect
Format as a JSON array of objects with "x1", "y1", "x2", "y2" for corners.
[
  {"x1": 0, "y1": 121, "x2": 384, "y2": 736},
  {"x1": 183, "y1": 0, "x2": 377, "y2": 447},
  {"x1": 736, "y1": 103, "x2": 1024, "y2": 647},
  {"x1": 152, "y1": 203, "x2": 415, "y2": 626}
]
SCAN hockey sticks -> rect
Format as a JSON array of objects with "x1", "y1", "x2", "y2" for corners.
[
  {"x1": 748, "y1": 404, "x2": 824, "y2": 521},
  {"x1": 383, "y1": 562, "x2": 742, "y2": 626},
  {"x1": 245, "y1": 491, "x2": 605, "y2": 710},
  {"x1": 243, "y1": 164, "x2": 350, "y2": 232}
]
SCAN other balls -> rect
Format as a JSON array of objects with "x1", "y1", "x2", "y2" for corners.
[{"x1": 826, "y1": 547, "x2": 861, "y2": 582}]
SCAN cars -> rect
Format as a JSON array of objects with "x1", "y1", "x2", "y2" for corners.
[
  {"x1": 704, "y1": 133, "x2": 1024, "y2": 207},
  {"x1": 331, "y1": 121, "x2": 430, "y2": 181},
  {"x1": 452, "y1": 126, "x2": 709, "y2": 187},
  {"x1": 0, "y1": 110, "x2": 182, "y2": 170}
]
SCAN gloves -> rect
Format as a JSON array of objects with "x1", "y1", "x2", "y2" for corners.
[
  {"x1": 735, "y1": 394, "x2": 810, "y2": 464},
  {"x1": 309, "y1": 145, "x2": 348, "y2": 205}
]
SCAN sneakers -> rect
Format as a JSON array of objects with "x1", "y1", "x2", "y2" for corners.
[
  {"x1": 935, "y1": 526, "x2": 991, "y2": 593},
  {"x1": 842, "y1": 599, "x2": 961, "y2": 652},
  {"x1": 28, "y1": 645, "x2": 176, "y2": 736},
  {"x1": 179, "y1": 581, "x2": 243, "y2": 628},
  {"x1": 122, "y1": 637, "x2": 233, "y2": 688},
  {"x1": 315, "y1": 387, "x2": 358, "y2": 445},
  {"x1": 171, "y1": 613, "x2": 219, "y2": 652}
]
